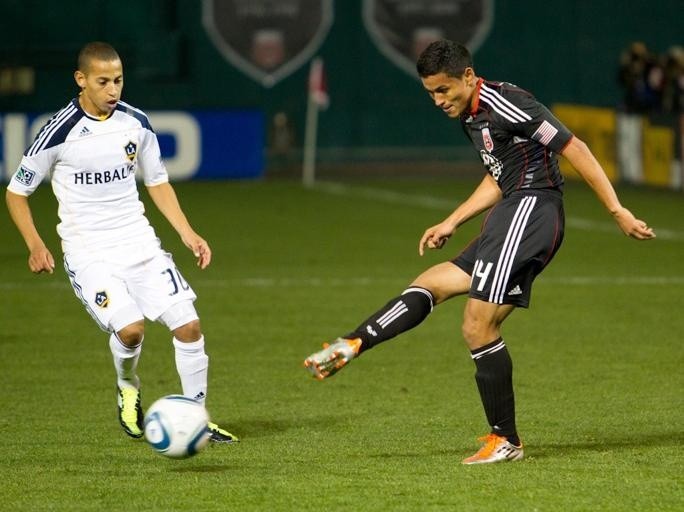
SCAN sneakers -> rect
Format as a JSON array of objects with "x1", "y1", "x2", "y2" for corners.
[
  {"x1": 117, "y1": 375, "x2": 143, "y2": 438},
  {"x1": 207, "y1": 422, "x2": 240, "y2": 443},
  {"x1": 460, "y1": 435, "x2": 524, "y2": 465},
  {"x1": 304, "y1": 337, "x2": 362, "y2": 381}
]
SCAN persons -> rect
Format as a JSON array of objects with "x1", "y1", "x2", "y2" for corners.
[
  {"x1": 303, "y1": 40, "x2": 657, "y2": 466},
  {"x1": 5, "y1": 41, "x2": 238, "y2": 443},
  {"x1": 617, "y1": 43, "x2": 684, "y2": 189}
]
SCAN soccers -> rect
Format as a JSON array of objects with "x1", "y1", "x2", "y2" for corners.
[{"x1": 143, "y1": 394, "x2": 210, "y2": 459}]
